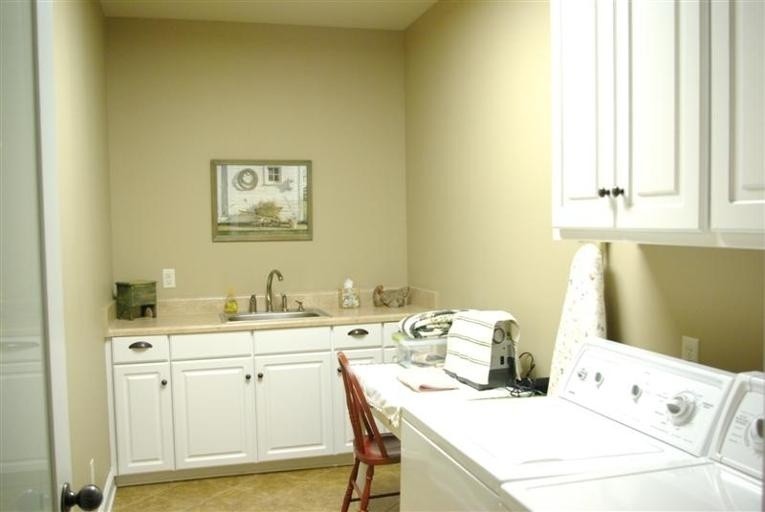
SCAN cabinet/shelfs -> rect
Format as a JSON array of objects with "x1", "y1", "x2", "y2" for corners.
[
  {"x1": 167, "y1": 328, "x2": 334, "y2": 469},
  {"x1": 336, "y1": 323, "x2": 399, "y2": 456},
  {"x1": 111, "y1": 335, "x2": 167, "y2": 476}
]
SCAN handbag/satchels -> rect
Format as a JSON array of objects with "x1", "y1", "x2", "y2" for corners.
[{"x1": 399, "y1": 309, "x2": 472, "y2": 340}]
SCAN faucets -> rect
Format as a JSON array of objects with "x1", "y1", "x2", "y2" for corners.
[{"x1": 266, "y1": 270, "x2": 283, "y2": 313}]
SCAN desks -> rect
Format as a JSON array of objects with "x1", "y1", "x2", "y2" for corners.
[{"x1": 347, "y1": 362, "x2": 517, "y2": 438}]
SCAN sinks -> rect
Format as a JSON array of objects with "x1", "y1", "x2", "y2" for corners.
[{"x1": 219, "y1": 309, "x2": 330, "y2": 323}]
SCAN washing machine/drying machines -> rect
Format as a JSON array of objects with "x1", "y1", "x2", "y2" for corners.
[
  {"x1": 499, "y1": 371, "x2": 764, "y2": 512},
  {"x1": 399, "y1": 336, "x2": 764, "y2": 512}
]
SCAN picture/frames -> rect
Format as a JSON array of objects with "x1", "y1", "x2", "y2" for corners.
[{"x1": 210, "y1": 157, "x2": 314, "y2": 244}]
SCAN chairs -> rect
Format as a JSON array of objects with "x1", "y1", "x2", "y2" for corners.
[{"x1": 336, "y1": 351, "x2": 401, "y2": 512}]
